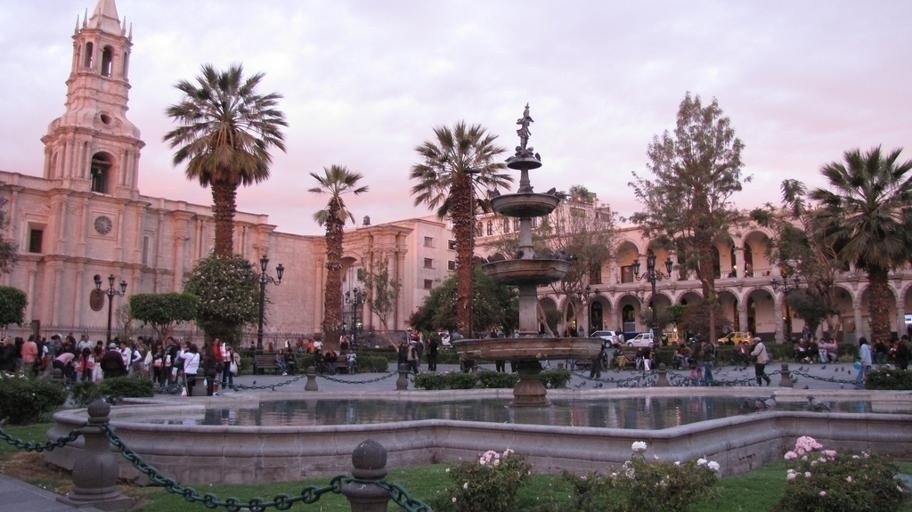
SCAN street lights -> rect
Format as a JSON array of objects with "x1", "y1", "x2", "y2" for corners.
[
  {"x1": 342, "y1": 285, "x2": 367, "y2": 344},
  {"x1": 241, "y1": 254, "x2": 286, "y2": 375},
  {"x1": 632, "y1": 247, "x2": 674, "y2": 349},
  {"x1": 769, "y1": 269, "x2": 803, "y2": 342},
  {"x1": 94, "y1": 272, "x2": 129, "y2": 350}
]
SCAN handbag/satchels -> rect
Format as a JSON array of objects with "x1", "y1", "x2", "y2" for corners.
[
  {"x1": 750, "y1": 355, "x2": 758, "y2": 361},
  {"x1": 230, "y1": 362, "x2": 238, "y2": 374}
]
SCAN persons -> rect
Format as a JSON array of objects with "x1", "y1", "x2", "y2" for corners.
[
  {"x1": 744, "y1": 263, "x2": 754, "y2": 279},
  {"x1": 727, "y1": 264, "x2": 737, "y2": 278},
  {"x1": 1, "y1": 334, "x2": 241, "y2": 398},
  {"x1": 515, "y1": 109, "x2": 536, "y2": 146},
  {"x1": 269, "y1": 332, "x2": 358, "y2": 374},
  {"x1": 249, "y1": 340, "x2": 256, "y2": 357},
  {"x1": 588, "y1": 393, "x2": 715, "y2": 430},
  {"x1": 588, "y1": 332, "x2": 605, "y2": 379},
  {"x1": 253, "y1": 398, "x2": 380, "y2": 427},
  {"x1": 398, "y1": 329, "x2": 438, "y2": 378},
  {"x1": 611, "y1": 327, "x2": 771, "y2": 388},
  {"x1": 859, "y1": 334, "x2": 911, "y2": 384},
  {"x1": 783, "y1": 331, "x2": 839, "y2": 361},
  {"x1": 494, "y1": 331, "x2": 506, "y2": 374}
]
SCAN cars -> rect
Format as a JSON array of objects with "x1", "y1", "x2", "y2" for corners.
[
  {"x1": 717, "y1": 331, "x2": 753, "y2": 346},
  {"x1": 625, "y1": 331, "x2": 654, "y2": 348},
  {"x1": 430, "y1": 330, "x2": 464, "y2": 346}
]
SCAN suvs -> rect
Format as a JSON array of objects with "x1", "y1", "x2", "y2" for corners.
[
  {"x1": 589, "y1": 329, "x2": 625, "y2": 349},
  {"x1": 360, "y1": 328, "x2": 412, "y2": 351}
]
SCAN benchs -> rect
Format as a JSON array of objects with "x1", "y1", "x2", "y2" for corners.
[
  {"x1": 575, "y1": 351, "x2": 650, "y2": 370},
  {"x1": 337, "y1": 356, "x2": 358, "y2": 373},
  {"x1": 253, "y1": 354, "x2": 296, "y2": 376}
]
